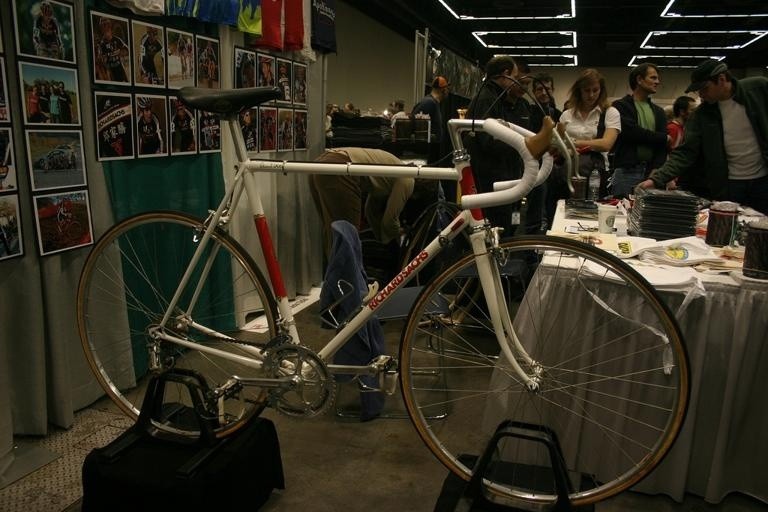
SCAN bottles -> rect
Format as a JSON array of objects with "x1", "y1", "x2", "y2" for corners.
[{"x1": 588, "y1": 168, "x2": 601, "y2": 203}]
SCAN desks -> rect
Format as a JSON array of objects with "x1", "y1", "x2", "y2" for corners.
[{"x1": 484, "y1": 199, "x2": 768, "y2": 505}]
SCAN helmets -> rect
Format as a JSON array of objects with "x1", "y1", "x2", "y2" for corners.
[
  {"x1": 138, "y1": 96, "x2": 153, "y2": 110},
  {"x1": 39, "y1": 3, "x2": 54, "y2": 17},
  {"x1": 98, "y1": 97, "x2": 131, "y2": 142}
]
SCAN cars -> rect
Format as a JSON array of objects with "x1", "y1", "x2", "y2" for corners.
[{"x1": 32, "y1": 143, "x2": 79, "y2": 170}]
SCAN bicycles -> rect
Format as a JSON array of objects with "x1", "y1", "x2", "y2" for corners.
[
  {"x1": 70, "y1": 80, "x2": 695, "y2": 503},
  {"x1": 36, "y1": 47, "x2": 65, "y2": 60},
  {"x1": 199, "y1": 56, "x2": 217, "y2": 86},
  {"x1": 178, "y1": 51, "x2": 195, "y2": 79},
  {"x1": 54, "y1": 199, "x2": 82, "y2": 241}
]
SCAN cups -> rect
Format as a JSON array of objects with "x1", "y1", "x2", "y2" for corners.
[
  {"x1": 570, "y1": 175, "x2": 588, "y2": 201},
  {"x1": 596, "y1": 204, "x2": 617, "y2": 235}
]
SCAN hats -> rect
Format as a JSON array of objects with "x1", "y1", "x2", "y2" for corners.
[
  {"x1": 684, "y1": 59, "x2": 729, "y2": 93},
  {"x1": 431, "y1": 76, "x2": 451, "y2": 89}
]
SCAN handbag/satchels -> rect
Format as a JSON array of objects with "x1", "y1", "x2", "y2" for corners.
[{"x1": 572, "y1": 150, "x2": 607, "y2": 181}]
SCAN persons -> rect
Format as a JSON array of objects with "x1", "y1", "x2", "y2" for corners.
[
  {"x1": 411, "y1": 76, "x2": 450, "y2": 154},
  {"x1": 0, "y1": 2, "x2": 307, "y2": 257},
  {"x1": 324, "y1": 98, "x2": 409, "y2": 147},
  {"x1": 463, "y1": 55, "x2": 565, "y2": 295},
  {"x1": 558, "y1": 63, "x2": 696, "y2": 199},
  {"x1": 637, "y1": 58, "x2": 767, "y2": 213},
  {"x1": 310, "y1": 147, "x2": 439, "y2": 266}
]
